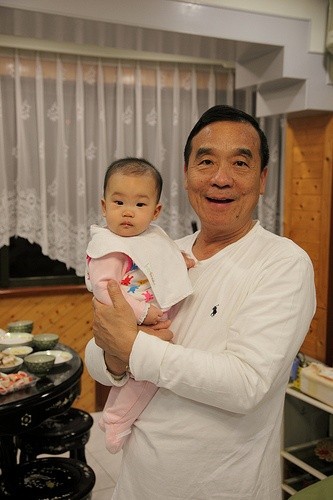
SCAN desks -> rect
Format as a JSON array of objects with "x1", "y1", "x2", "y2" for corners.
[{"x1": 0, "y1": 342, "x2": 84, "y2": 500}]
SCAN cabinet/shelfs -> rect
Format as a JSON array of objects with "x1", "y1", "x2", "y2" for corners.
[{"x1": 280, "y1": 378, "x2": 333, "y2": 500}]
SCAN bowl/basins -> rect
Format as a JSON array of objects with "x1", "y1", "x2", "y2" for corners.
[
  {"x1": 8, "y1": 371, "x2": 40, "y2": 391},
  {"x1": 0, "y1": 333, "x2": 33, "y2": 350},
  {"x1": 24, "y1": 354, "x2": 56, "y2": 376},
  {"x1": 8, "y1": 320, "x2": 33, "y2": 334},
  {"x1": 32, "y1": 333, "x2": 59, "y2": 350}
]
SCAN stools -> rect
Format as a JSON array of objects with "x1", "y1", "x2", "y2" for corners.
[
  {"x1": 0, "y1": 457, "x2": 96, "y2": 500},
  {"x1": 15, "y1": 407, "x2": 94, "y2": 464}
]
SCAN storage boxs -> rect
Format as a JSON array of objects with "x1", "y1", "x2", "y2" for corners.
[{"x1": 302, "y1": 362, "x2": 333, "y2": 407}]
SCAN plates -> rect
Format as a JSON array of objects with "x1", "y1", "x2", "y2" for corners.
[
  {"x1": 3, "y1": 346, "x2": 33, "y2": 357},
  {"x1": 0, "y1": 357, "x2": 23, "y2": 372},
  {"x1": 31, "y1": 350, "x2": 73, "y2": 367}
]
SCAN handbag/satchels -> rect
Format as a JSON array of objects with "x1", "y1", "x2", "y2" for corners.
[{"x1": 288, "y1": 350, "x2": 305, "y2": 383}]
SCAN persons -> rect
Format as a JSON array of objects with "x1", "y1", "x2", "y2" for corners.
[
  {"x1": 84, "y1": 158, "x2": 195, "y2": 455},
  {"x1": 108, "y1": 105, "x2": 316, "y2": 500}
]
